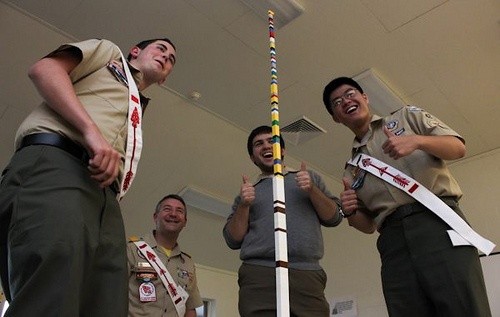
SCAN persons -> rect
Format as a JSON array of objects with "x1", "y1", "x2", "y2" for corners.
[
  {"x1": 222, "y1": 125, "x2": 343, "y2": 317},
  {"x1": 127, "y1": 194, "x2": 204, "y2": 317},
  {"x1": 323, "y1": 77, "x2": 496, "y2": 317},
  {"x1": 0, "y1": 38, "x2": 176, "y2": 317}
]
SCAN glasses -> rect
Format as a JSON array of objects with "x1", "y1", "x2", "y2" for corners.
[{"x1": 331, "y1": 89, "x2": 360, "y2": 108}]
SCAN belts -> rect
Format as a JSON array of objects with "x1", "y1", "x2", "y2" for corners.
[
  {"x1": 380, "y1": 198, "x2": 459, "y2": 228},
  {"x1": 17, "y1": 132, "x2": 89, "y2": 164}
]
